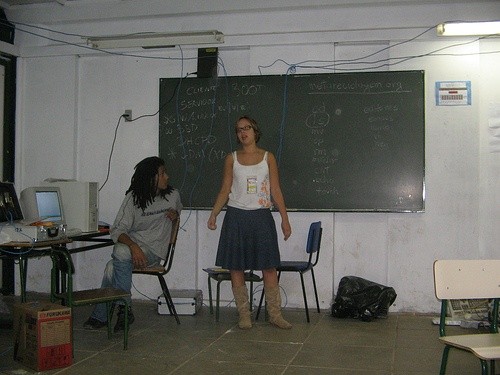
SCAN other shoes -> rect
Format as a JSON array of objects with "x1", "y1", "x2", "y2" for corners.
[
  {"x1": 114, "y1": 306, "x2": 135, "y2": 335},
  {"x1": 83, "y1": 317, "x2": 107, "y2": 330}
]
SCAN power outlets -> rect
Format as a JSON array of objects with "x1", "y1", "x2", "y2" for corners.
[{"x1": 124, "y1": 109, "x2": 133, "y2": 123}]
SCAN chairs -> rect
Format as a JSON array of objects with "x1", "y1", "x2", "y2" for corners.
[
  {"x1": 253, "y1": 220, "x2": 323, "y2": 324},
  {"x1": 201, "y1": 267, "x2": 264, "y2": 324},
  {"x1": 131, "y1": 214, "x2": 183, "y2": 325},
  {"x1": 50, "y1": 248, "x2": 131, "y2": 350},
  {"x1": 433, "y1": 258, "x2": 500, "y2": 375}
]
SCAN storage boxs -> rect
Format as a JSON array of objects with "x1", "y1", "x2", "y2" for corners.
[
  {"x1": 157, "y1": 288, "x2": 204, "y2": 317},
  {"x1": 12, "y1": 299, "x2": 72, "y2": 373}
]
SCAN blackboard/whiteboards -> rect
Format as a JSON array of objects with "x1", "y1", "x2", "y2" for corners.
[{"x1": 159, "y1": 70, "x2": 425, "y2": 212}]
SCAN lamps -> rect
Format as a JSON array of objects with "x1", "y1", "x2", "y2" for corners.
[
  {"x1": 435, "y1": 19, "x2": 500, "y2": 37},
  {"x1": 86, "y1": 29, "x2": 226, "y2": 49}
]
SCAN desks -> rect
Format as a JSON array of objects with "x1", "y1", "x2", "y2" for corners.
[{"x1": 0, "y1": 226, "x2": 115, "y2": 361}]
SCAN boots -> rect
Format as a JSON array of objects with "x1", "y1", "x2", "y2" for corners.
[
  {"x1": 264, "y1": 285, "x2": 293, "y2": 329},
  {"x1": 231, "y1": 284, "x2": 254, "y2": 330}
]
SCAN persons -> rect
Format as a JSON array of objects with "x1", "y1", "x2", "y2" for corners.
[
  {"x1": 83, "y1": 156, "x2": 185, "y2": 335},
  {"x1": 206, "y1": 116, "x2": 292, "y2": 331}
]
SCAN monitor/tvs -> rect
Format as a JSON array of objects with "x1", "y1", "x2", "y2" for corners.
[{"x1": 18, "y1": 185, "x2": 65, "y2": 234}]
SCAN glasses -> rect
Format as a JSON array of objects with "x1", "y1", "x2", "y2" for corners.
[{"x1": 237, "y1": 125, "x2": 252, "y2": 134}]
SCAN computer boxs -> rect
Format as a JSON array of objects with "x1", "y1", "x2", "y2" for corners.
[{"x1": 41, "y1": 180, "x2": 98, "y2": 232}]
